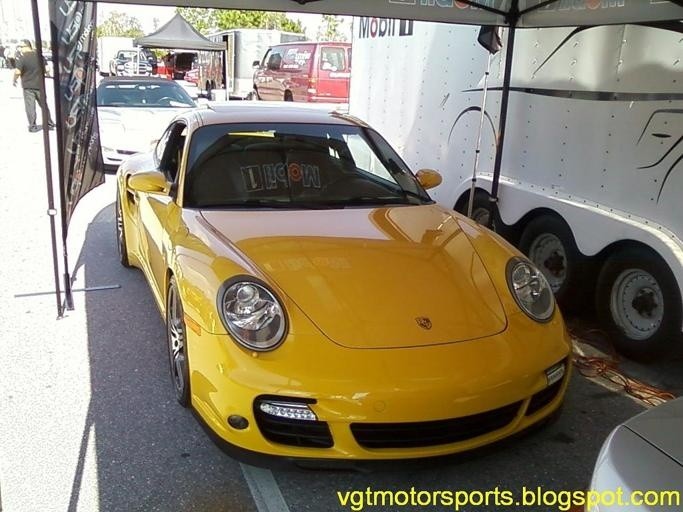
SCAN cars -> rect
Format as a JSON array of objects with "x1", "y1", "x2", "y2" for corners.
[
  {"x1": 109, "y1": 50, "x2": 198, "y2": 86},
  {"x1": 0, "y1": 39, "x2": 54, "y2": 78},
  {"x1": 585, "y1": 397, "x2": 683, "y2": 512},
  {"x1": 96, "y1": 77, "x2": 199, "y2": 175}
]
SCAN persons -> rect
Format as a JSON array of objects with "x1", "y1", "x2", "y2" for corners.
[{"x1": 12, "y1": 38, "x2": 55, "y2": 133}]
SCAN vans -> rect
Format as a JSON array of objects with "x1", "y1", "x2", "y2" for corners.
[{"x1": 253, "y1": 41, "x2": 351, "y2": 103}]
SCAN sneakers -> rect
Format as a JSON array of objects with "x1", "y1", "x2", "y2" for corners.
[{"x1": 25, "y1": 122, "x2": 55, "y2": 134}]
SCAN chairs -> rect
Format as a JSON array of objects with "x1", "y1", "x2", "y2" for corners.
[
  {"x1": 190, "y1": 141, "x2": 357, "y2": 203},
  {"x1": 109, "y1": 86, "x2": 168, "y2": 104}
]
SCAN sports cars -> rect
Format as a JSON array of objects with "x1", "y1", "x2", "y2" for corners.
[{"x1": 115, "y1": 107, "x2": 573, "y2": 472}]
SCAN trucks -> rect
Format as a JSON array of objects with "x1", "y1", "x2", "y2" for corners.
[
  {"x1": 96, "y1": 37, "x2": 133, "y2": 76},
  {"x1": 198, "y1": 29, "x2": 317, "y2": 100}
]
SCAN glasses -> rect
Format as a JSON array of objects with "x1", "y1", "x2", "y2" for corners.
[{"x1": 17, "y1": 45, "x2": 28, "y2": 49}]
